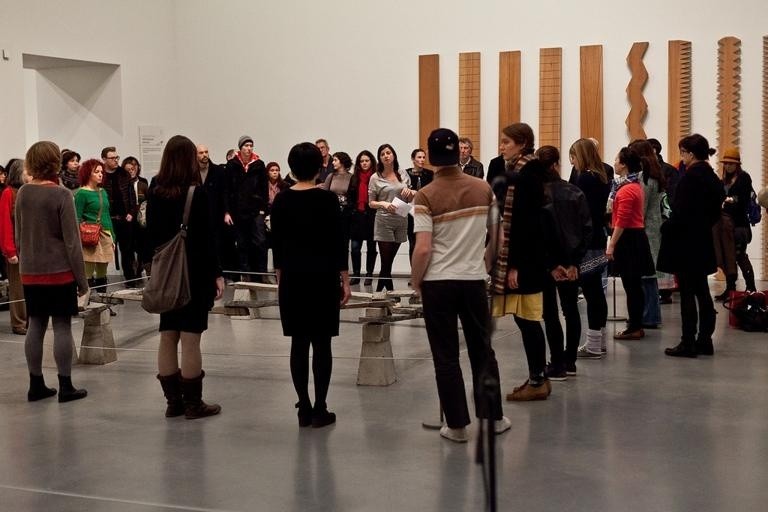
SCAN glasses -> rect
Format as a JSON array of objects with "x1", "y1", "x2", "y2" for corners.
[
  {"x1": 105, "y1": 156, "x2": 120, "y2": 160},
  {"x1": 681, "y1": 149, "x2": 688, "y2": 154}
]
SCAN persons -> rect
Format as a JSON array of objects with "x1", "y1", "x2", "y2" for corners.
[
  {"x1": 59, "y1": 151, "x2": 81, "y2": 193},
  {"x1": 714, "y1": 148, "x2": 756, "y2": 301},
  {"x1": 225, "y1": 135, "x2": 268, "y2": 284},
  {"x1": 124, "y1": 163, "x2": 148, "y2": 283},
  {"x1": 569, "y1": 138, "x2": 609, "y2": 360},
  {"x1": 74, "y1": 159, "x2": 117, "y2": 317},
  {"x1": 488, "y1": 123, "x2": 552, "y2": 403},
  {"x1": 271, "y1": 142, "x2": 352, "y2": 427},
  {"x1": 486, "y1": 154, "x2": 512, "y2": 183},
  {"x1": 649, "y1": 138, "x2": 678, "y2": 304},
  {"x1": 226, "y1": 148, "x2": 236, "y2": 161},
  {"x1": 122, "y1": 156, "x2": 148, "y2": 184},
  {"x1": 267, "y1": 162, "x2": 290, "y2": 214},
  {"x1": 100, "y1": 147, "x2": 137, "y2": 287},
  {"x1": 316, "y1": 138, "x2": 334, "y2": 190},
  {"x1": 146, "y1": 135, "x2": 225, "y2": 420},
  {"x1": 14, "y1": 140, "x2": 88, "y2": 403},
  {"x1": 656, "y1": 134, "x2": 723, "y2": 358},
  {"x1": 605, "y1": 147, "x2": 645, "y2": 341},
  {"x1": 457, "y1": 137, "x2": 484, "y2": 179},
  {"x1": 411, "y1": 128, "x2": 512, "y2": 443},
  {"x1": 405, "y1": 149, "x2": 434, "y2": 285},
  {"x1": 346, "y1": 150, "x2": 377, "y2": 286},
  {"x1": 629, "y1": 138, "x2": 678, "y2": 329},
  {"x1": 0, "y1": 165, "x2": 7, "y2": 195},
  {"x1": 535, "y1": 145, "x2": 586, "y2": 381},
  {"x1": 370, "y1": 144, "x2": 417, "y2": 293},
  {"x1": 321, "y1": 152, "x2": 352, "y2": 208},
  {"x1": 570, "y1": 138, "x2": 614, "y2": 204},
  {"x1": 0, "y1": 160, "x2": 33, "y2": 335},
  {"x1": 196, "y1": 144, "x2": 217, "y2": 189}
]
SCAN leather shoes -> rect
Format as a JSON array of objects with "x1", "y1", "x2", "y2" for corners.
[
  {"x1": 13, "y1": 326, "x2": 27, "y2": 335},
  {"x1": 665, "y1": 346, "x2": 695, "y2": 358},
  {"x1": 696, "y1": 347, "x2": 713, "y2": 355}
]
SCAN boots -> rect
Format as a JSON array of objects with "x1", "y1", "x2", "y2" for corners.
[
  {"x1": 364, "y1": 251, "x2": 378, "y2": 285},
  {"x1": 578, "y1": 330, "x2": 602, "y2": 359},
  {"x1": 601, "y1": 328, "x2": 607, "y2": 356},
  {"x1": 312, "y1": 403, "x2": 336, "y2": 427},
  {"x1": 715, "y1": 273, "x2": 735, "y2": 298},
  {"x1": 295, "y1": 400, "x2": 313, "y2": 427},
  {"x1": 94, "y1": 277, "x2": 116, "y2": 316},
  {"x1": 157, "y1": 368, "x2": 185, "y2": 417},
  {"x1": 58, "y1": 373, "x2": 87, "y2": 403},
  {"x1": 349, "y1": 251, "x2": 362, "y2": 285},
  {"x1": 181, "y1": 370, "x2": 221, "y2": 417},
  {"x1": 28, "y1": 374, "x2": 57, "y2": 402}
]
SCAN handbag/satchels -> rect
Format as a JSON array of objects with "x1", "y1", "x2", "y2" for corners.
[
  {"x1": 142, "y1": 186, "x2": 196, "y2": 313},
  {"x1": 79, "y1": 187, "x2": 102, "y2": 247},
  {"x1": 749, "y1": 186, "x2": 761, "y2": 226},
  {"x1": 722, "y1": 295, "x2": 768, "y2": 332}
]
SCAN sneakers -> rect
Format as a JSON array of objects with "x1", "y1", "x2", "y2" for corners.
[
  {"x1": 440, "y1": 426, "x2": 469, "y2": 442},
  {"x1": 514, "y1": 377, "x2": 552, "y2": 395},
  {"x1": 546, "y1": 363, "x2": 568, "y2": 380},
  {"x1": 641, "y1": 329, "x2": 645, "y2": 338},
  {"x1": 613, "y1": 330, "x2": 641, "y2": 340},
  {"x1": 563, "y1": 363, "x2": 577, "y2": 375},
  {"x1": 495, "y1": 417, "x2": 511, "y2": 433},
  {"x1": 506, "y1": 383, "x2": 548, "y2": 402}
]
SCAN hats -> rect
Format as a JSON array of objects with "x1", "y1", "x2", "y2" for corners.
[
  {"x1": 238, "y1": 136, "x2": 253, "y2": 149},
  {"x1": 428, "y1": 128, "x2": 460, "y2": 167},
  {"x1": 720, "y1": 151, "x2": 741, "y2": 164}
]
